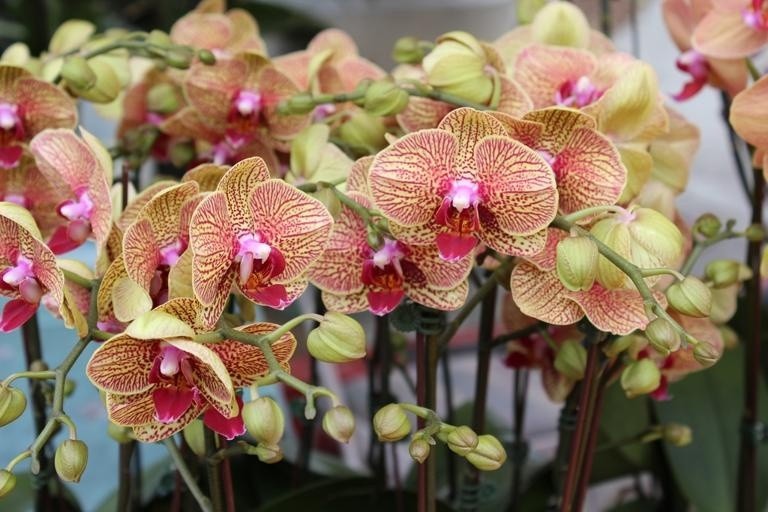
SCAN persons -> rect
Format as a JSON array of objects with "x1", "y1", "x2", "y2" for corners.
[
  {"x1": 498, "y1": 1, "x2": 768, "y2": 512},
  {"x1": 1, "y1": 230, "x2": 184, "y2": 510}
]
